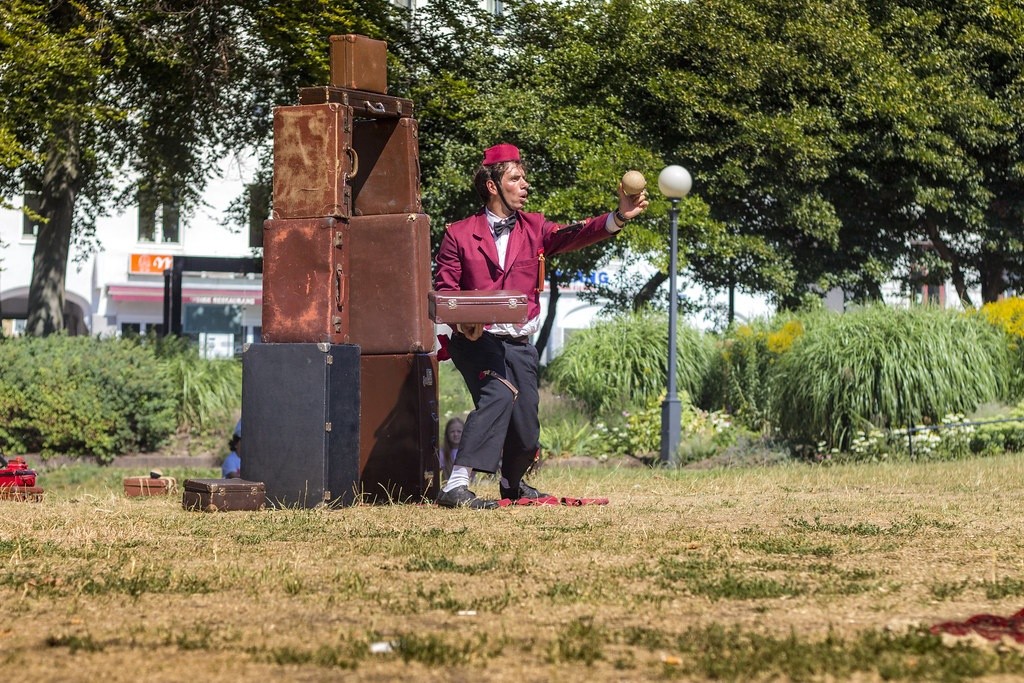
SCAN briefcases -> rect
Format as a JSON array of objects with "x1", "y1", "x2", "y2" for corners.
[
  {"x1": 353, "y1": 118, "x2": 422, "y2": 216},
  {"x1": 329, "y1": 34, "x2": 387, "y2": 95},
  {"x1": 124, "y1": 476, "x2": 177, "y2": 496},
  {"x1": 273, "y1": 103, "x2": 358, "y2": 219},
  {"x1": 241, "y1": 343, "x2": 361, "y2": 509},
  {"x1": 182, "y1": 478, "x2": 267, "y2": 512},
  {"x1": 360, "y1": 355, "x2": 440, "y2": 502},
  {"x1": 298, "y1": 87, "x2": 414, "y2": 118},
  {"x1": 0, "y1": 486, "x2": 43, "y2": 502},
  {"x1": 348, "y1": 213, "x2": 434, "y2": 353},
  {"x1": 428, "y1": 291, "x2": 529, "y2": 326},
  {"x1": 0, "y1": 469, "x2": 37, "y2": 487},
  {"x1": 261, "y1": 217, "x2": 350, "y2": 344}
]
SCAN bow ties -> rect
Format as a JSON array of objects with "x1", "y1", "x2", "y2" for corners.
[{"x1": 494, "y1": 216, "x2": 516, "y2": 237}]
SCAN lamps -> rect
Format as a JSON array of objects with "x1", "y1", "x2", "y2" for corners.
[{"x1": 659, "y1": 164, "x2": 693, "y2": 209}]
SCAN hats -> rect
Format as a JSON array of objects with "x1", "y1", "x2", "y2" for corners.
[
  {"x1": 483, "y1": 144, "x2": 520, "y2": 165},
  {"x1": 235, "y1": 420, "x2": 241, "y2": 438}
]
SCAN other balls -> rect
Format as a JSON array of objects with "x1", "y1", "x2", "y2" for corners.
[{"x1": 623, "y1": 170, "x2": 645, "y2": 195}]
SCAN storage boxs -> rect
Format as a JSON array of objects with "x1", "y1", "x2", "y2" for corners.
[
  {"x1": 242, "y1": 342, "x2": 362, "y2": 511},
  {"x1": 349, "y1": 213, "x2": 436, "y2": 353},
  {"x1": 181, "y1": 478, "x2": 265, "y2": 514},
  {"x1": 329, "y1": 34, "x2": 389, "y2": 94},
  {"x1": 360, "y1": 354, "x2": 441, "y2": 504},
  {"x1": 123, "y1": 476, "x2": 177, "y2": 498},
  {"x1": 428, "y1": 289, "x2": 529, "y2": 324},
  {"x1": 0, "y1": 457, "x2": 45, "y2": 503}
]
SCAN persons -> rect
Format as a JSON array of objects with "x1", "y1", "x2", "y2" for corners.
[
  {"x1": 221, "y1": 434, "x2": 240, "y2": 480},
  {"x1": 431, "y1": 144, "x2": 650, "y2": 510},
  {"x1": 438, "y1": 417, "x2": 476, "y2": 485}
]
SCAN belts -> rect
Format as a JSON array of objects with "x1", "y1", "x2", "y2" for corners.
[{"x1": 494, "y1": 334, "x2": 529, "y2": 344}]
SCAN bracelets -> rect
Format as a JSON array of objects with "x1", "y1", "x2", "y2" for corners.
[{"x1": 615, "y1": 208, "x2": 631, "y2": 223}]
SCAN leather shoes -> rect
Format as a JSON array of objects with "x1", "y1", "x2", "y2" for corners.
[
  {"x1": 436, "y1": 485, "x2": 498, "y2": 510},
  {"x1": 500, "y1": 480, "x2": 549, "y2": 500}
]
